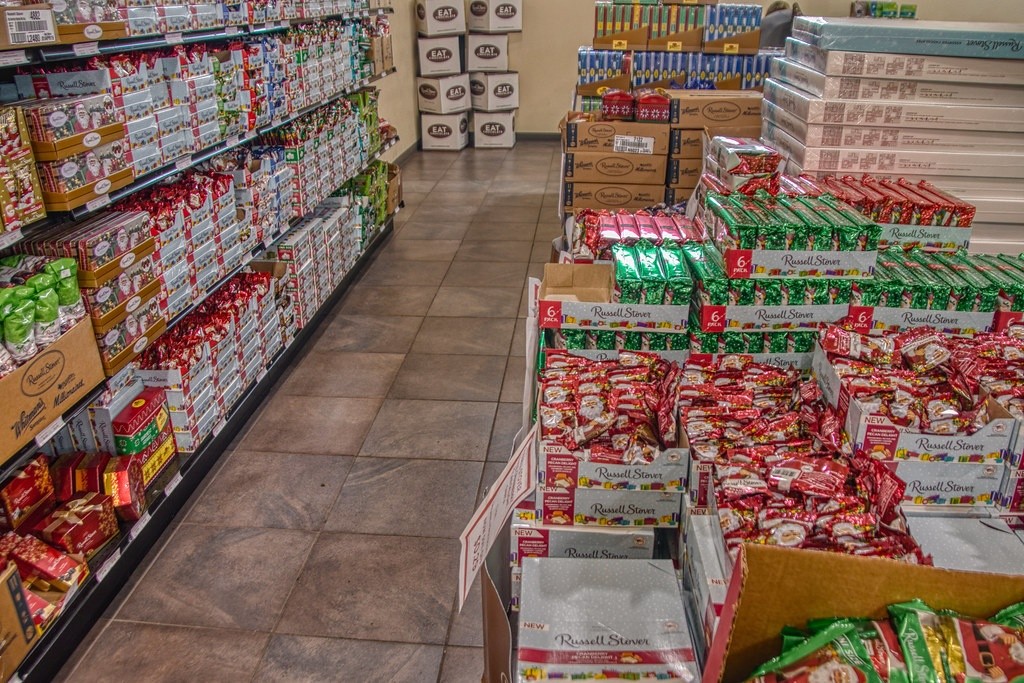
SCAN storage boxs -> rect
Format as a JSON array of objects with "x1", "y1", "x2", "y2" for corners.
[
  {"x1": 415, "y1": 0, "x2": 1024, "y2": 683},
  {"x1": 0, "y1": 0, "x2": 409, "y2": 682}
]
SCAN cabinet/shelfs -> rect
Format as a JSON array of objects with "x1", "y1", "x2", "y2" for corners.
[{"x1": 0, "y1": 0, "x2": 395, "y2": 683}]
